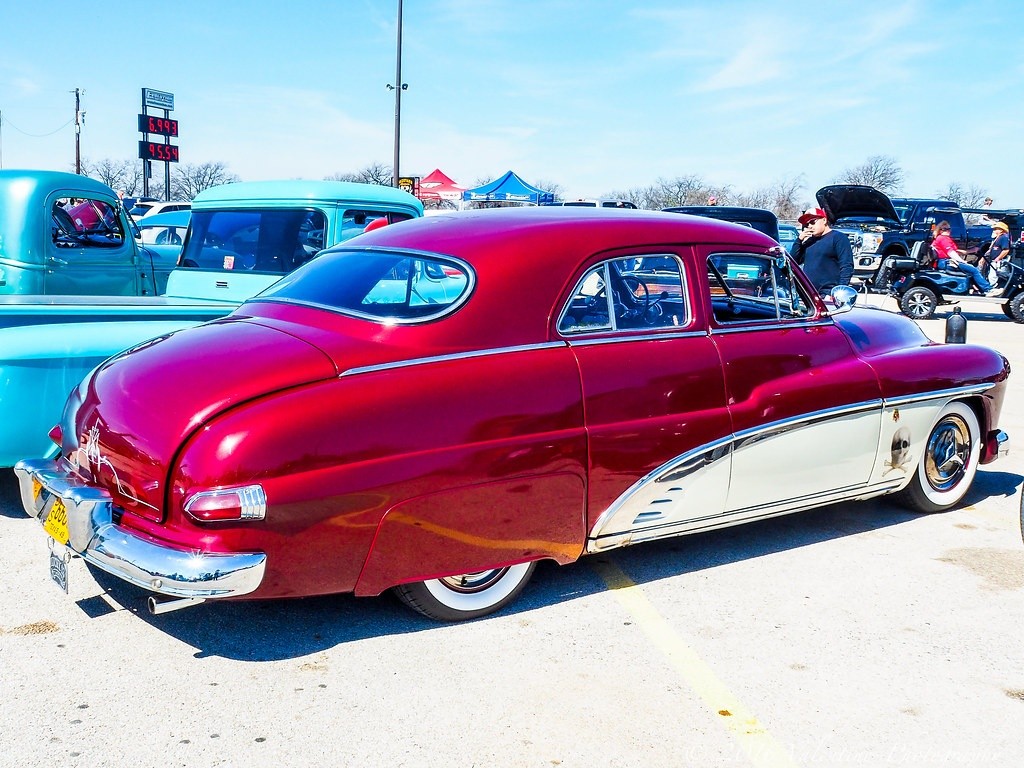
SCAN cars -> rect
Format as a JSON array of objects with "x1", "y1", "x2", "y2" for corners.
[
  {"x1": 0, "y1": 170, "x2": 468, "y2": 319},
  {"x1": 14, "y1": 206, "x2": 1010, "y2": 622}
]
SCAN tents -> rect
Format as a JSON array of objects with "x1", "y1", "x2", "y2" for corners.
[{"x1": 419, "y1": 168, "x2": 554, "y2": 211}]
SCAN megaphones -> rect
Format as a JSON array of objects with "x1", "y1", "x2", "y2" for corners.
[
  {"x1": 402, "y1": 83, "x2": 408, "y2": 91},
  {"x1": 386, "y1": 84, "x2": 395, "y2": 91}
]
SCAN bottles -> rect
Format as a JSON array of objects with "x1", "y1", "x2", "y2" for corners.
[{"x1": 944, "y1": 307, "x2": 967, "y2": 344}]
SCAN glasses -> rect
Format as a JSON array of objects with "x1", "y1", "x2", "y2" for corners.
[
  {"x1": 940, "y1": 228, "x2": 951, "y2": 231},
  {"x1": 805, "y1": 218, "x2": 823, "y2": 228}
]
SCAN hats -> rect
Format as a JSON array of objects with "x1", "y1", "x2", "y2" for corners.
[
  {"x1": 798, "y1": 208, "x2": 827, "y2": 224},
  {"x1": 991, "y1": 222, "x2": 1009, "y2": 234},
  {"x1": 985, "y1": 197, "x2": 993, "y2": 201}
]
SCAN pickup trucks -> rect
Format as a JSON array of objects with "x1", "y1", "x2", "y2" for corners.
[
  {"x1": 0, "y1": 180, "x2": 424, "y2": 473},
  {"x1": 816, "y1": 184, "x2": 991, "y2": 277},
  {"x1": 540, "y1": 199, "x2": 800, "y2": 296}
]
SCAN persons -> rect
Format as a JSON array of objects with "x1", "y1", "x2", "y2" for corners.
[
  {"x1": 977, "y1": 222, "x2": 1009, "y2": 279},
  {"x1": 981, "y1": 197, "x2": 993, "y2": 221},
  {"x1": 930, "y1": 221, "x2": 1004, "y2": 297},
  {"x1": 790, "y1": 207, "x2": 855, "y2": 299}
]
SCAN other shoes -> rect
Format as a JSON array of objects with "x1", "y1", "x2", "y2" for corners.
[{"x1": 986, "y1": 288, "x2": 1004, "y2": 297}]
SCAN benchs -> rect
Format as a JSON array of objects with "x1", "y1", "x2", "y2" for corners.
[{"x1": 911, "y1": 241, "x2": 967, "y2": 278}]
